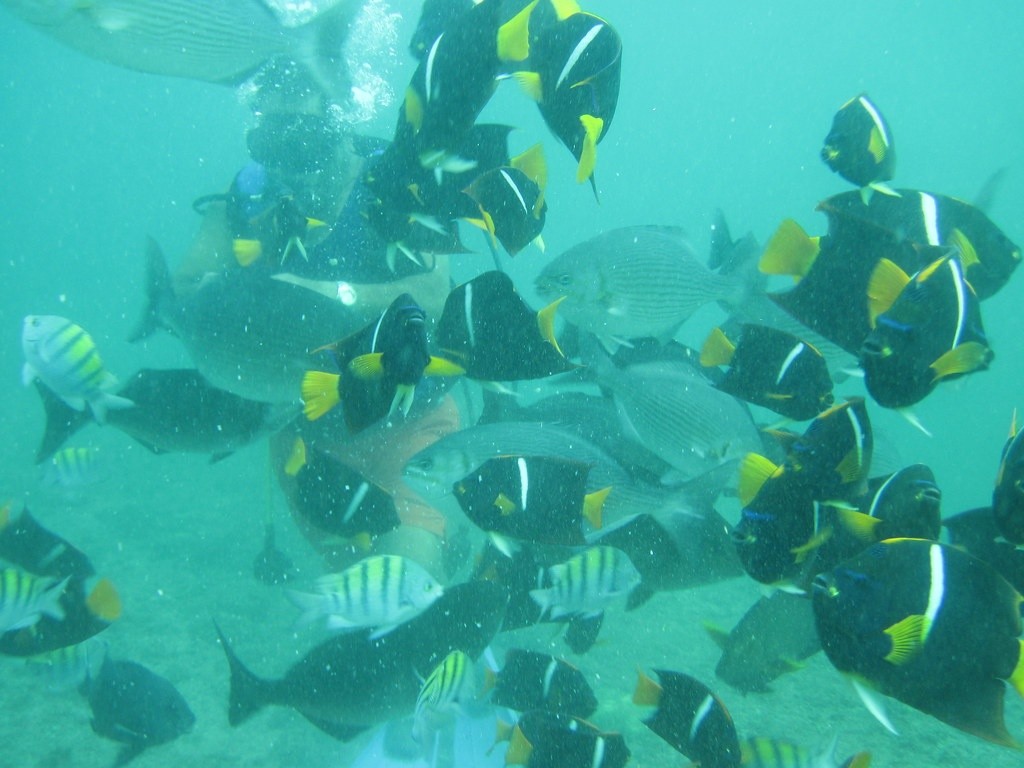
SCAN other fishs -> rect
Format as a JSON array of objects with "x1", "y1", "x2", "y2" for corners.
[{"x1": 0, "y1": 0, "x2": 1022, "y2": 766}]
